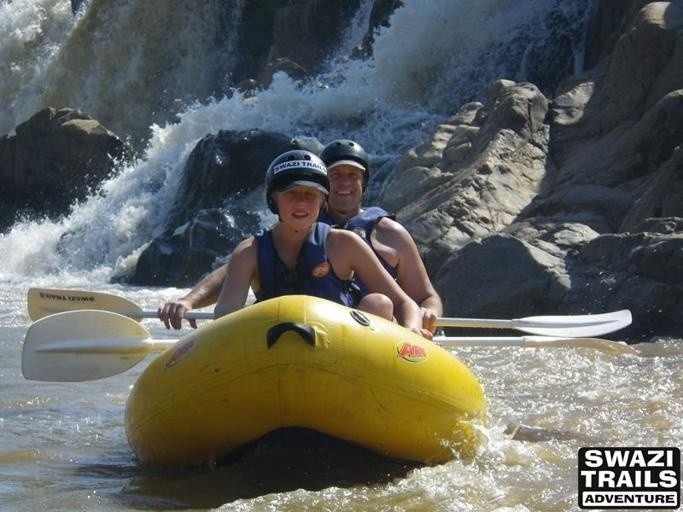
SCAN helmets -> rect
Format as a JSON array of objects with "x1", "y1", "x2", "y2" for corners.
[
  {"x1": 265, "y1": 149, "x2": 330, "y2": 214},
  {"x1": 320, "y1": 139, "x2": 370, "y2": 193}
]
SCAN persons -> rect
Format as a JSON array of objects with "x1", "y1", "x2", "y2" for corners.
[
  {"x1": 213, "y1": 151, "x2": 433, "y2": 342},
  {"x1": 158, "y1": 140, "x2": 444, "y2": 337}
]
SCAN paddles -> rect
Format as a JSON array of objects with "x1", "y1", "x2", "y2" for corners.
[
  {"x1": 21, "y1": 310, "x2": 639, "y2": 383},
  {"x1": 26, "y1": 286, "x2": 634, "y2": 340}
]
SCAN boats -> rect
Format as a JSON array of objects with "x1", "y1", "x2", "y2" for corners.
[{"x1": 123, "y1": 292, "x2": 492, "y2": 474}]
